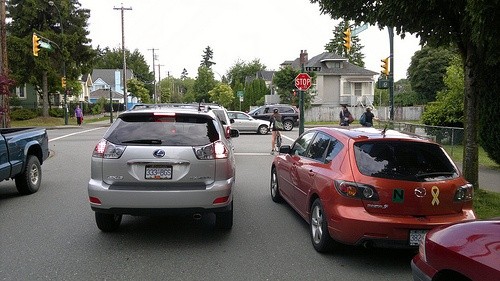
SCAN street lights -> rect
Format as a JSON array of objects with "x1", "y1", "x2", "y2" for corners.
[{"x1": 49, "y1": 0, "x2": 69, "y2": 125}]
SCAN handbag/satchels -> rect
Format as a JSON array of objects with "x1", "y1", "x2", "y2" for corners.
[
  {"x1": 274, "y1": 120, "x2": 283, "y2": 129},
  {"x1": 349, "y1": 115, "x2": 354, "y2": 123},
  {"x1": 360, "y1": 114, "x2": 366, "y2": 124}
]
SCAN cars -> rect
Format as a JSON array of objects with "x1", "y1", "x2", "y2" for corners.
[
  {"x1": 130, "y1": 104, "x2": 235, "y2": 140},
  {"x1": 87, "y1": 108, "x2": 239, "y2": 232},
  {"x1": 410, "y1": 220, "x2": 500, "y2": 281},
  {"x1": 270, "y1": 127, "x2": 477, "y2": 253},
  {"x1": 226, "y1": 111, "x2": 273, "y2": 135}
]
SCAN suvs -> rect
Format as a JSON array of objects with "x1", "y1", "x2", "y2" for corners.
[{"x1": 247, "y1": 104, "x2": 301, "y2": 131}]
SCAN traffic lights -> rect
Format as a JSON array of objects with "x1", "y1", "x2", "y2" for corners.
[
  {"x1": 343, "y1": 28, "x2": 351, "y2": 52},
  {"x1": 33, "y1": 35, "x2": 41, "y2": 57},
  {"x1": 61, "y1": 77, "x2": 67, "y2": 87},
  {"x1": 381, "y1": 58, "x2": 389, "y2": 75}
]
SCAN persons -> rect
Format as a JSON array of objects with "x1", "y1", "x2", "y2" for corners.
[
  {"x1": 338, "y1": 105, "x2": 351, "y2": 126},
  {"x1": 362, "y1": 107, "x2": 375, "y2": 127},
  {"x1": 294, "y1": 104, "x2": 299, "y2": 114},
  {"x1": 263, "y1": 107, "x2": 268, "y2": 114},
  {"x1": 268, "y1": 108, "x2": 283, "y2": 155},
  {"x1": 74, "y1": 105, "x2": 83, "y2": 126}
]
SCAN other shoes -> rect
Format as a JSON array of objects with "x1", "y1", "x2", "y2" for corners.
[{"x1": 272, "y1": 150, "x2": 274, "y2": 154}]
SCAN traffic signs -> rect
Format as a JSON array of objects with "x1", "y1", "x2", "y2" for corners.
[{"x1": 294, "y1": 73, "x2": 312, "y2": 91}]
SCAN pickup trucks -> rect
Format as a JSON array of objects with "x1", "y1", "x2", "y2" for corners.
[{"x1": 0, "y1": 126, "x2": 51, "y2": 195}]
